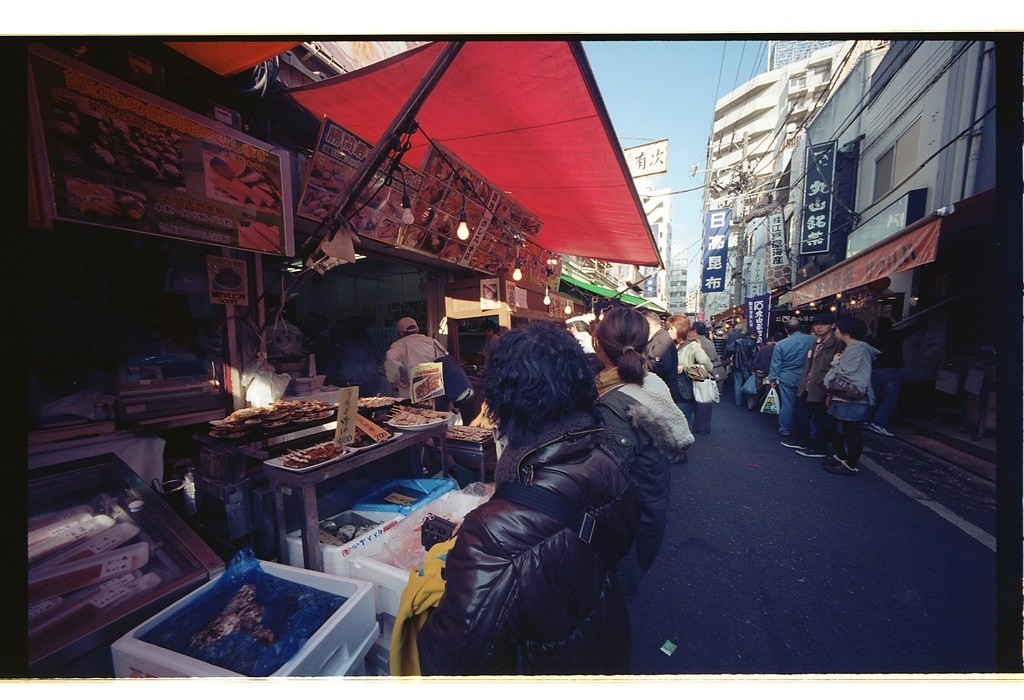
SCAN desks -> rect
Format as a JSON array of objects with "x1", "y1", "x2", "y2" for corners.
[{"x1": 185, "y1": 426, "x2": 452, "y2": 571}]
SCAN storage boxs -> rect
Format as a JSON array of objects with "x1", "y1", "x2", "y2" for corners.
[{"x1": 112, "y1": 479, "x2": 489, "y2": 677}]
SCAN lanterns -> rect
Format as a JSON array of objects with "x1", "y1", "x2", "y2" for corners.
[{"x1": 870, "y1": 277, "x2": 891, "y2": 292}]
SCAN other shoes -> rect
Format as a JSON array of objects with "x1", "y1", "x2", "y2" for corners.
[
  {"x1": 867, "y1": 423, "x2": 893, "y2": 436},
  {"x1": 824, "y1": 459, "x2": 859, "y2": 475},
  {"x1": 796, "y1": 447, "x2": 826, "y2": 457},
  {"x1": 780, "y1": 439, "x2": 810, "y2": 449},
  {"x1": 818, "y1": 454, "x2": 847, "y2": 467},
  {"x1": 689, "y1": 426, "x2": 710, "y2": 435}
]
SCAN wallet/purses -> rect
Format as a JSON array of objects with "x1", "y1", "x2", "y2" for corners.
[{"x1": 420, "y1": 512, "x2": 461, "y2": 551}]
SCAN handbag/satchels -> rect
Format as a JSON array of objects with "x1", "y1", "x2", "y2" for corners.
[
  {"x1": 740, "y1": 374, "x2": 757, "y2": 395},
  {"x1": 760, "y1": 387, "x2": 780, "y2": 414},
  {"x1": 816, "y1": 369, "x2": 868, "y2": 401},
  {"x1": 693, "y1": 365, "x2": 720, "y2": 404},
  {"x1": 433, "y1": 340, "x2": 474, "y2": 402}
]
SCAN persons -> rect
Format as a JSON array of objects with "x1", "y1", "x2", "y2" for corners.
[
  {"x1": 266, "y1": 309, "x2": 302, "y2": 376},
  {"x1": 564, "y1": 308, "x2": 720, "y2": 435},
  {"x1": 590, "y1": 308, "x2": 694, "y2": 613},
  {"x1": 417, "y1": 322, "x2": 643, "y2": 676},
  {"x1": 863, "y1": 310, "x2": 925, "y2": 436},
  {"x1": 708, "y1": 318, "x2": 817, "y2": 435},
  {"x1": 384, "y1": 317, "x2": 457, "y2": 477},
  {"x1": 821, "y1": 318, "x2": 882, "y2": 475},
  {"x1": 780, "y1": 311, "x2": 847, "y2": 457},
  {"x1": 477, "y1": 320, "x2": 499, "y2": 360}
]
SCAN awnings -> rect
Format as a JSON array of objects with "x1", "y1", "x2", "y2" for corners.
[
  {"x1": 287, "y1": 41, "x2": 673, "y2": 316},
  {"x1": 711, "y1": 188, "x2": 996, "y2": 321}
]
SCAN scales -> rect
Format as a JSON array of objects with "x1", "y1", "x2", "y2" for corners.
[{"x1": 116, "y1": 355, "x2": 215, "y2": 424}]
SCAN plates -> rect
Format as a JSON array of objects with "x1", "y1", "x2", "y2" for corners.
[
  {"x1": 209, "y1": 405, "x2": 335, "y2": 438},
  {"x1": 474, "y1": 183, "x2": 491, "y2": 205},
  {"x1": 428, "y1": 155, "x2": 453, "y2": 182},
  {"x1": 263, "y1": 443, "x2": 360, "y2": 473},
  {"x1": 388, "y1": 413, "x2": 452, "y2": 431},
  {"x1": 328, "y1": 432, "x2": 403, "y2": 451},
  {"x1": 434, "y1": 215, "x2": 454, "y2": 235},
  {"x1": 442, "y1": 191, "x2": 465, "y2": 216}
]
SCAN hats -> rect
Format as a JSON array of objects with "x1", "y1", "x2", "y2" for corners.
[
  {"x1": 396, "y1": 317, "x2": 420, "y2": 332},
  {"x1": 812, "y1": 314, "x2": 833, "y2": 323}
]
